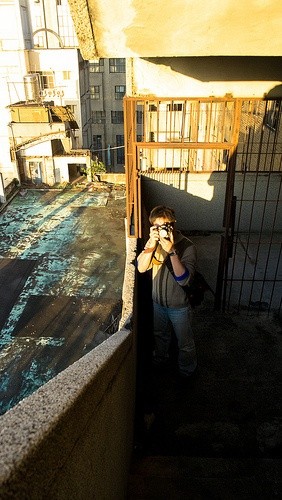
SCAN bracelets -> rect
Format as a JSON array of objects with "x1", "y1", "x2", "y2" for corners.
[{"x1": 169, "y1": 249, "x2": 177, "y2": 256}]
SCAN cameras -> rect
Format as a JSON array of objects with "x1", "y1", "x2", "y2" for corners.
[{"x1": 157, "y1": 224, "x2": 173, "y2": 238}]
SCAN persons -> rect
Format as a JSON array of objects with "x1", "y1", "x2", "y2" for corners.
[{"x1": 137, "y1": 205, "x2": 199, "y2": 380}]
